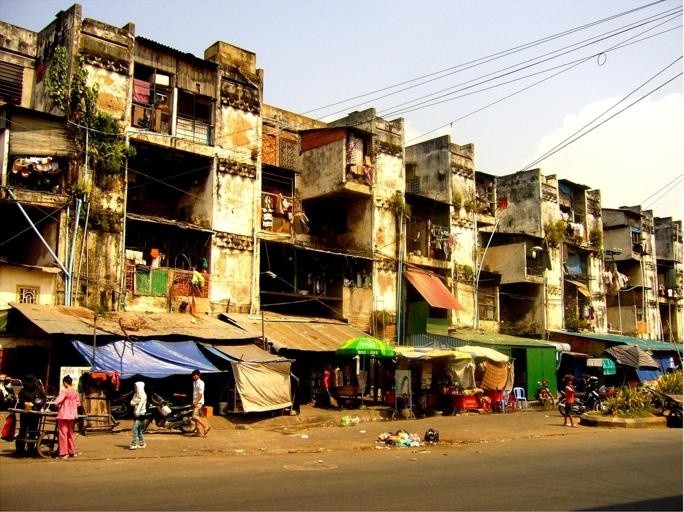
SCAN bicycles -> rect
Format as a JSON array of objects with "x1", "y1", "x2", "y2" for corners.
[{"x1": 536, "y1": 380, "x2": 554, "y2": 406}]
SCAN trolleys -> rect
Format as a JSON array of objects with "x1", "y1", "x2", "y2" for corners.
[{"x1": 8, "y1": 407, "x2": 119, "y2": 459}]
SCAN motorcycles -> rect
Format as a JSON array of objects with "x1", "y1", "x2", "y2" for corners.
[
  {"x1": 556, "y1": 376, "x2": 603, "y2": 416},
  {"x1": 110, "y1": 391, "x2": 196, "y2": 433}
]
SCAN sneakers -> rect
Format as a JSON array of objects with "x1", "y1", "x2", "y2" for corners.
[
  {"x1": 60, "y1": 454, "x2": 78, "y2": 458},
  {"x1": 130, "y1": 442, "x2": 146, "y2": 449},
  {"x1": 193, "y1": 428, "x2": 210, "y2": 436}
]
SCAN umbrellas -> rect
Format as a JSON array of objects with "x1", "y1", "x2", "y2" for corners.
[
  {"x1": 603, "y1": 344, "x2": 660, "y2": 368},
  {"x1": 336, "y1": 337, "x2": 395, "y2": 405}
]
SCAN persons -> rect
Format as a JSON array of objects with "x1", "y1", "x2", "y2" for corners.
[
  {"x1": 129, "y1": 381, "x2": 147, "y2": 450},
  {"x1": 191, "y1": 370, "x2": 211, "y2": 436},
  {"x1": 562, "y1": 374, "x2": 575, "y2": 427},
  {"x1": 15, "y1": 375, "x2": 45, "y2": 457},
  {"x1": 54, "y1": 375, "x2": 81, "y2": 459}
]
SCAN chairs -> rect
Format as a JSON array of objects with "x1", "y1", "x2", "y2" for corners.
[{"x1": 513, "y1": 387, "x2": 527, "y2": 413}]
